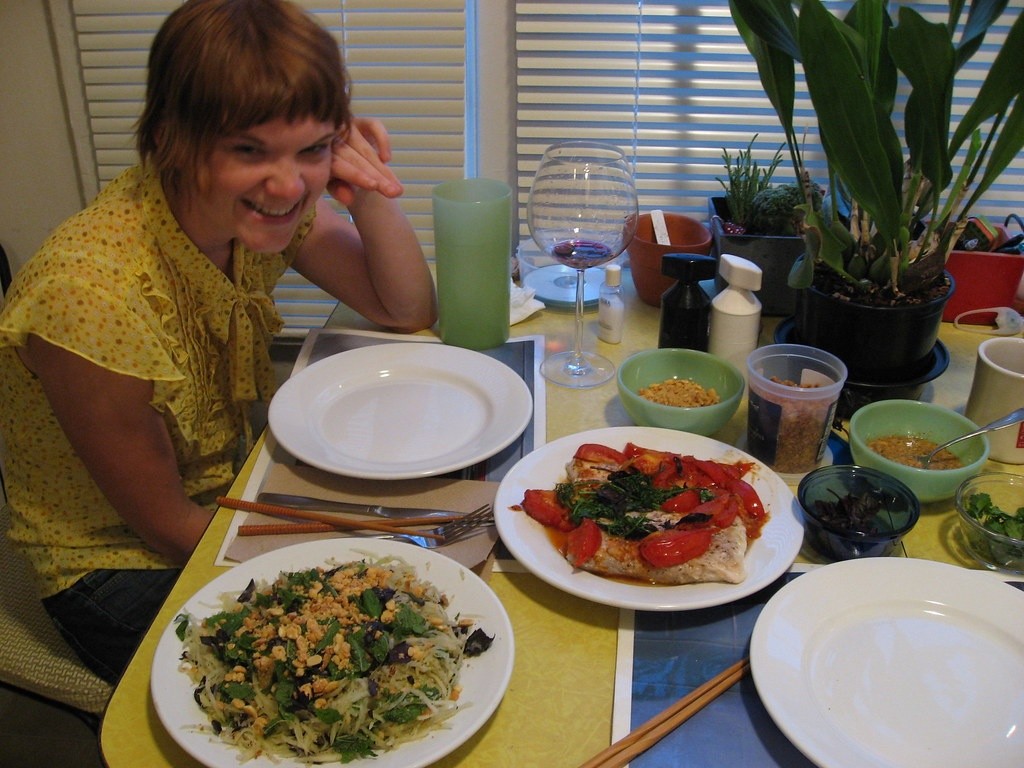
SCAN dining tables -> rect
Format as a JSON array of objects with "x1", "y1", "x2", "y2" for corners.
[{"x1": 94, "y1": 257, "x2": 1024, "y2": 768}]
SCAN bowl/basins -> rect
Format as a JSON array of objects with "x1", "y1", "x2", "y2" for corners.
[
  {"x1": 849, "y1": 400, "x2": 990, "y2": 504},
  {"x1": 517, "y1": 237, "x2": 601, "y2": 314},
  {"x1": 796, "y1": 463, "x2": 921, "y2": 562},
  {"x1": 954, "y1": 471, "x2": 1024, "y2": 576},
  {"x1": 616, "y1": 346, "x2": 745, "y2": 436}
]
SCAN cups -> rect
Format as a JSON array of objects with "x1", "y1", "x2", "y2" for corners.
[
  {"x1": 628, "y1": 212, "x2": 712, "y2": 307},
  {"x1": 430, "y1": 178, "x2": 511, "y2": 347},
  {"x1": 746, "y1": 344, "x2": 847, "y2": 472},
  {"x1": 964, "y1": 336, "x2": 1024, "y2": 465}
]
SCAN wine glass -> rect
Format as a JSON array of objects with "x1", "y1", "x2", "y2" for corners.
[{"x1": 526, "y1": 141, "x2": 638, "y2": 388}]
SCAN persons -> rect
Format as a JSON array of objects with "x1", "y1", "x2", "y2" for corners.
[{"x1": 0, "y1": 0, "x2": 437, "y2": 685}]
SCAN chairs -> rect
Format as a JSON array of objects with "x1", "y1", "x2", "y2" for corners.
[{"x1": 0, "y1": 235, "x2": 112, "y2": 768}]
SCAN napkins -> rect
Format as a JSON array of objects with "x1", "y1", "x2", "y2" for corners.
[
  {"x1": 510, "y1": 276, "x2": 547, "y2": 327},
  {"x1": 220, "y1": 468, "x2": 508, "y2": 583}
]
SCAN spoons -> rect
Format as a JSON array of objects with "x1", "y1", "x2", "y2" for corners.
[{"x1": 916, "y1": 406, "x2": 1024, "y2": 468}]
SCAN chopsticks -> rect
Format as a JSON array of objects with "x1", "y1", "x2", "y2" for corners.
[
  {"x1": 577, "y1": 659, "x2": 749, "y2": 768},
  {"x1": 216, "y1": 496, "x2": 464, "y2": 539}
]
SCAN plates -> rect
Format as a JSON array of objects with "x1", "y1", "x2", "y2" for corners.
[
  {"x1": 266, "y1": 342, "x2": 533, "y2": 479},
  {"x1": 495, "y1": 426, "x2": 804, "y2": 612},
  {"x1": 749, "y1": 556, "x2": 1024, "y2": 768},
  {"x1": 151, "y1": 536, "x2": 513, "y2": 767}
]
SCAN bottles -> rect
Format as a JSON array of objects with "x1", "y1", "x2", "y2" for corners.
[{"x1": 597, "y1": 264, "x2": 624, "y2": 344}]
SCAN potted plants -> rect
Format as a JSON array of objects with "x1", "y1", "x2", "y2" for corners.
[
  {"x1": 726, "y1": 0, "x2": 1024, "y2": 370},
  {"x1": 707, "y1": 129, "x2": 808, "y2": 316}
]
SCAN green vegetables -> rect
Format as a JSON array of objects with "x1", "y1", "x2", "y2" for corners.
[
  {"x1": 963, "y1": 493, "x2": 1024, "y2": 571},
  {"x1": 553, "y1": 463, "x2": 716, "y2": 542},
  {"x1": 174, "y1": 560, "x2": 495, "y2": 764}
]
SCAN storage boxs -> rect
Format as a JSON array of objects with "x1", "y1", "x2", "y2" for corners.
[{"x1": 941, "y1": 217, "x2": 1024, "y2": 325}]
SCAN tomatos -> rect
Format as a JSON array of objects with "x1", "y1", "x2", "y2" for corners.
[
  {"x1": 522, "y1": 489, "x2": 576, "y2": 532},
  {"x1": 565, "y1": 443, "x2": 770, "y2": 568}
]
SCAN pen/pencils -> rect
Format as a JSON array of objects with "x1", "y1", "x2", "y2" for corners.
[
  {"x1": 993, "y1": 234, "x2": 1024, "y2": 254},
  {"x1": 962, "y1": 238, "x2": 980, "y2": 251}
]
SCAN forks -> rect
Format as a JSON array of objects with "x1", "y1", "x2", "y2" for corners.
[{"x1": 362, "y1": 503, "x2": 492, "y2": 547}]
